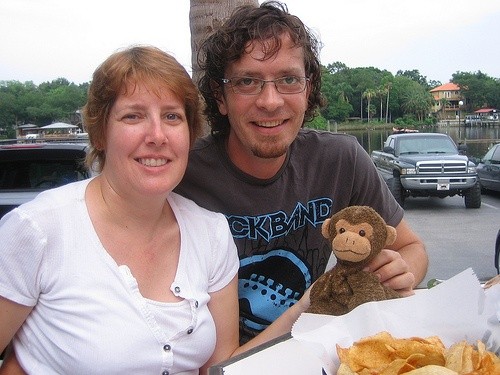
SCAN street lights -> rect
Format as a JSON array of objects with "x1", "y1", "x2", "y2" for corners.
[{"x1": 458, "y1": 100, "x2": 464, "y2": 145}]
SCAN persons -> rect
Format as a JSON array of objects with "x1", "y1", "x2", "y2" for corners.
[
  {"x1": 174, "y1": 1, "x2": 428, "y2": 345},
  {"x1": 0, "y1": 46, "x2": 320, "y2": 375}
]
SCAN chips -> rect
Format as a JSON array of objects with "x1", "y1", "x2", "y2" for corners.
[{"x1": 334, "y1": 331, "x2": 500, "y2": 375}]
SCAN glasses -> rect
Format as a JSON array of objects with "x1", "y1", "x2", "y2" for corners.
[{"x1": 221, "y1": 76, "x2": 310, "y2": 96}]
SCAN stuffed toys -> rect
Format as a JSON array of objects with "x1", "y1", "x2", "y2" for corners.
[{"x1": 306, "y1": 206, "x2": 398, "y2": 316}]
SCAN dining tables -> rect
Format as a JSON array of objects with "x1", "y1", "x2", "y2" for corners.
[{"x1": 208, "y1": 284, "x2": 500, "y2": 375}]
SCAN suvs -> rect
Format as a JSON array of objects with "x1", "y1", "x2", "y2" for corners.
[{"x1": 0, "y1": 139, "x2": 97, "y2": 219}]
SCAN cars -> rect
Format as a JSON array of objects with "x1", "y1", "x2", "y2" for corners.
[{"x1": 476, "y1": 143, "x2": 500, "y2": 195}]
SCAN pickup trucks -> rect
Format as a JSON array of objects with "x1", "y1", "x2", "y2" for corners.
[{"x1": 370, "y1": 131, "x2": 483, "y2": 210}]
